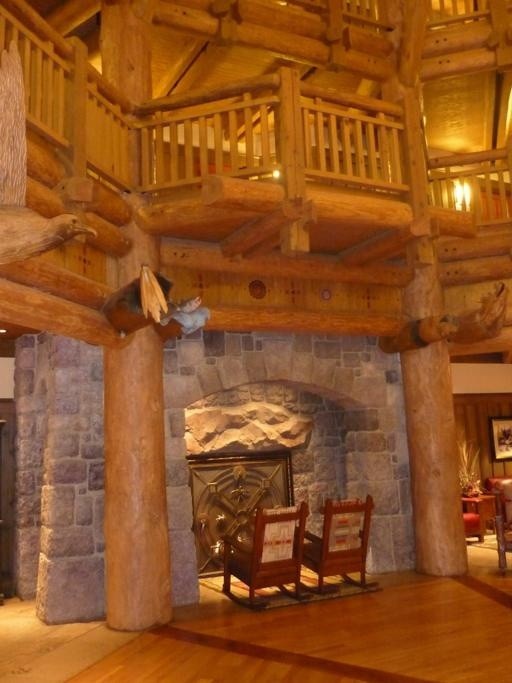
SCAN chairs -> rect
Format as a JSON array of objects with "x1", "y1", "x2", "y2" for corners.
[{"x1": 220, "y1": 493, "x2": 374, "y2": 605}]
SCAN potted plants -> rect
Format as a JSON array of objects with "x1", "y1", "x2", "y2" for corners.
[{"x1": 456, "y1": 431, "x2": 483, "y2": 497}]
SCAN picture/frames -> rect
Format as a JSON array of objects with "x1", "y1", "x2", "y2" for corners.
[{"x1": 486, "y1": 414, "x2": 512, "y2": 460}]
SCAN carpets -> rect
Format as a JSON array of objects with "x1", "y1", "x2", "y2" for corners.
[{"x1": 194, "y1": 560, "x2": 387, "y2": 611}]
